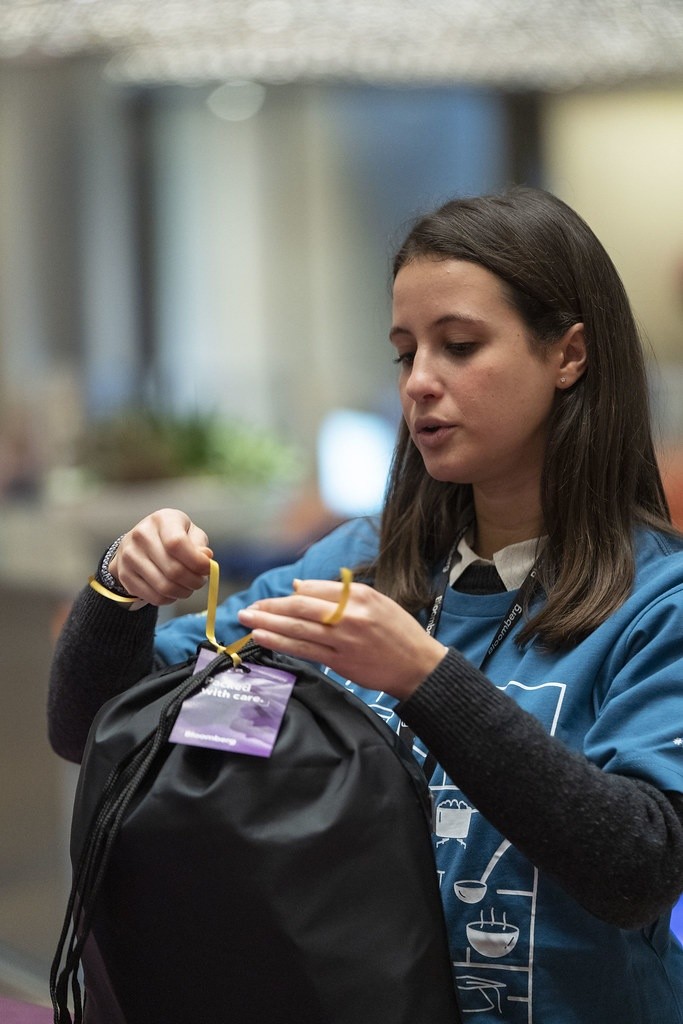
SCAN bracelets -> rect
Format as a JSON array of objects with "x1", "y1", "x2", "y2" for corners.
[{"x1": 100, "y1": 532, "x2": 127, "y2": 596}]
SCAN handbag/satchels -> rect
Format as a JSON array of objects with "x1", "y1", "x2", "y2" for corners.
[{"x1": 49, "y1": 640, "x2": 463, "y2": 1024}]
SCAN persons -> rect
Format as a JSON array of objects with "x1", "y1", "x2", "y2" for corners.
[{"x1": 49, "y1": 188, "x2": 683, "y2": 1024}]
